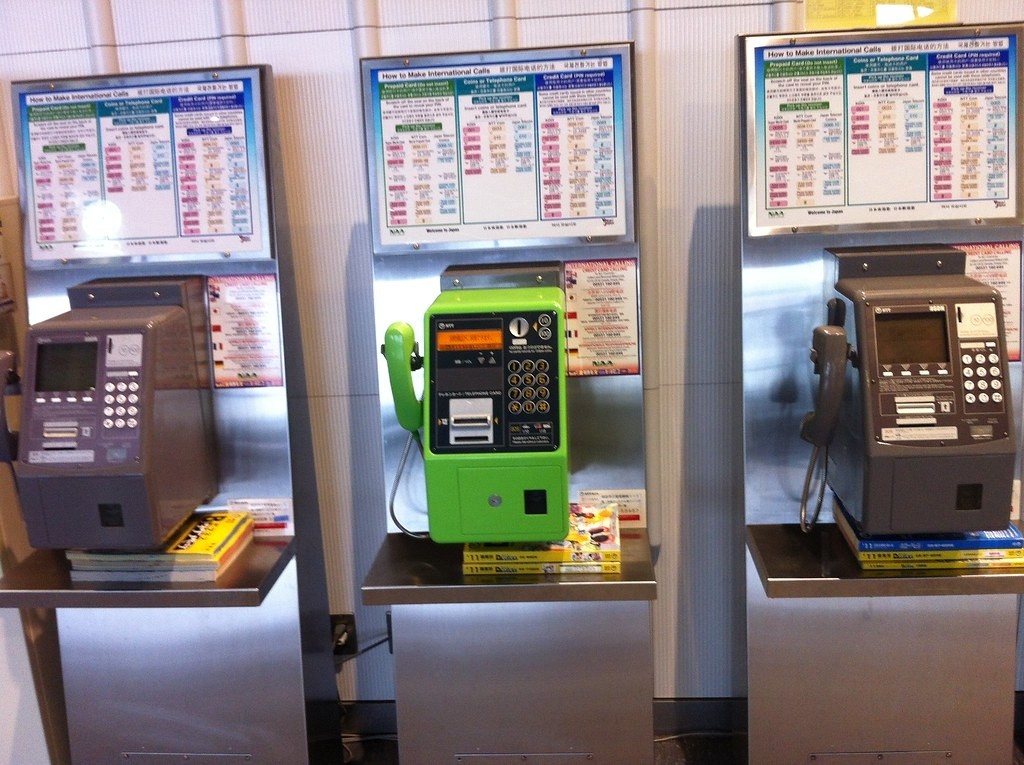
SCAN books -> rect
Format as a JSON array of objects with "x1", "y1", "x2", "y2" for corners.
[
  {"x1": 463, "y1": 502, "x2": 620, "y2": 573},
  {"x1": 67, "y1": 512, "x2": 255, "y2": 582},
  {"x1": 832, "y1": 496, "x2": 1024, "y2": 571}
]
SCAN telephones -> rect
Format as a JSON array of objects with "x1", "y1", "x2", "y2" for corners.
[
  {"x1": 380, "y1": 260, "x2": 570, "y2": 544},
  {"x1": 799, "y1": 244, "x2": 1018, "y2": 534},
  {"x1": 0, "y1": 274, "x2": 220, "y2": 551}
]
